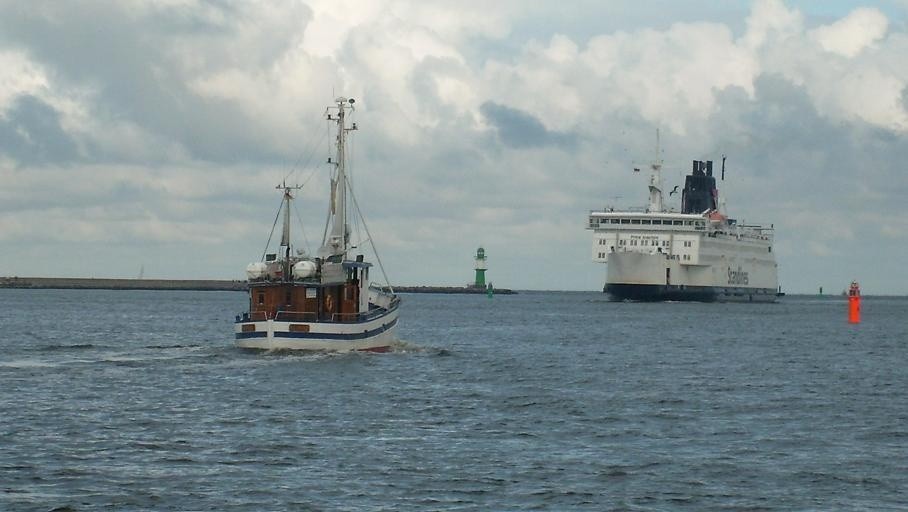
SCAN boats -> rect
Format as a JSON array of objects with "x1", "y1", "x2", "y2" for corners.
[{"x1": 232, "y1": 96, "x2": 403, "y2": 354}]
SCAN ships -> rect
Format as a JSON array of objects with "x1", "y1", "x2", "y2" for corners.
[{"x1": 586, "y1": 124, "x2": 779, "y2": 303}]
